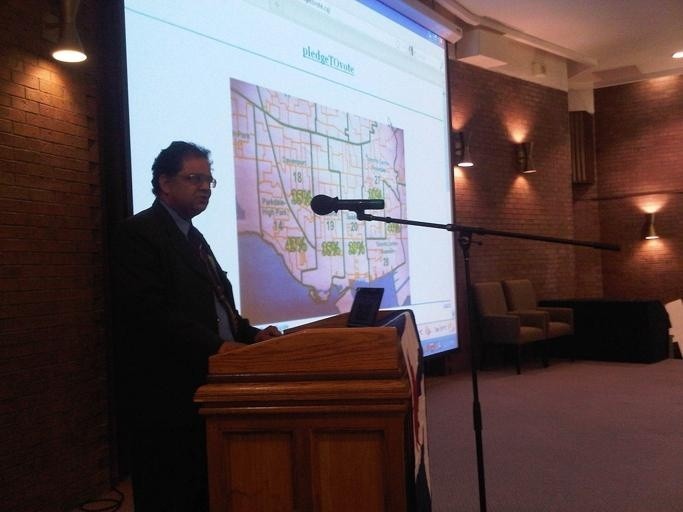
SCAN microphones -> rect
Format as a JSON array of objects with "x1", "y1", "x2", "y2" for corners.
[{"x1": 311, "y1": 195, "x2": 384, "y2": 215}]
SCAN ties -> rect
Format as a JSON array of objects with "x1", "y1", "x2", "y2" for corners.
[{"x1": 190, "y1": 230, "x2": 239, "y2": 335}]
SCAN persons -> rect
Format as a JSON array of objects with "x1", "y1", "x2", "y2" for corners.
[{"x1": 104, "y1": 141, "x2": 281, "y2": 511}]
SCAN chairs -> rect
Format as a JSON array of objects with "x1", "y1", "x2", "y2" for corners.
[
  {"x1": 502, "y1": 279, "x2": 574, "y2": 367},
  {"x1": 472, "y1": 282, "x2": 548, "y2": 375}
]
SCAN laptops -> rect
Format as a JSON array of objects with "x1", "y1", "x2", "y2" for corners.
[{"x1": 346, "y1": 288, "x2": 384, "y2": 327}]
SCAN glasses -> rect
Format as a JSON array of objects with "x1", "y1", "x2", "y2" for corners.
[{"x1": 173, "y1": 171, "x2": 218, "y2": 189}]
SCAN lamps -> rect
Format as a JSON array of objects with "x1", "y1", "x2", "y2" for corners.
[
  {"x1": 521, "y1": 141, "x2": 536, "y2": 174},
  {"x1": 644, "y1": 214, "x2": 659, "y2": 239},
  {"x1": 49, "y1": 0, "x2": 88, "y2": 63},
  {"x1": 457, "y1": 131, "x2": 474, "y2": 167}
]
